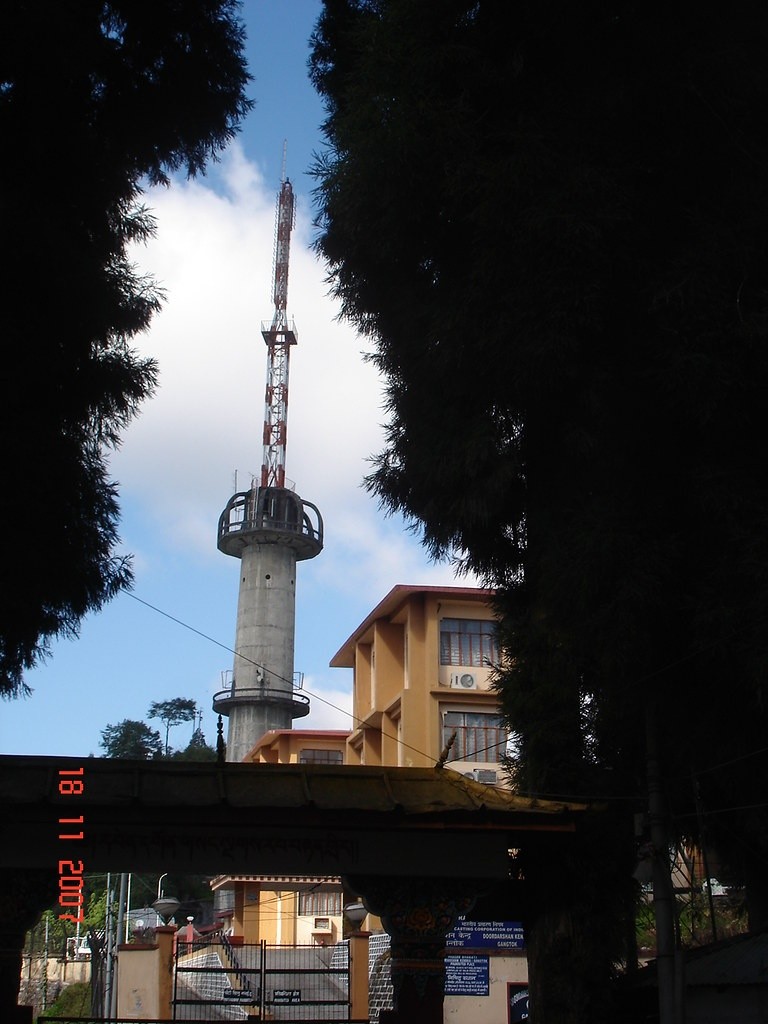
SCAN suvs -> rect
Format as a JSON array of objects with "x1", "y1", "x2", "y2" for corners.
[{"x1": 77, "y1": 929, "x2": 115, "y2": 959}]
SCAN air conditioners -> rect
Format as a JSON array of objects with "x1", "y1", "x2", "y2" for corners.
[
  {"x1": 451, "y1": 672, "x2": 477, "y2": 689},
  {"x1": 459, "y1": 769, "x2": 497, "y2": 784}
]
